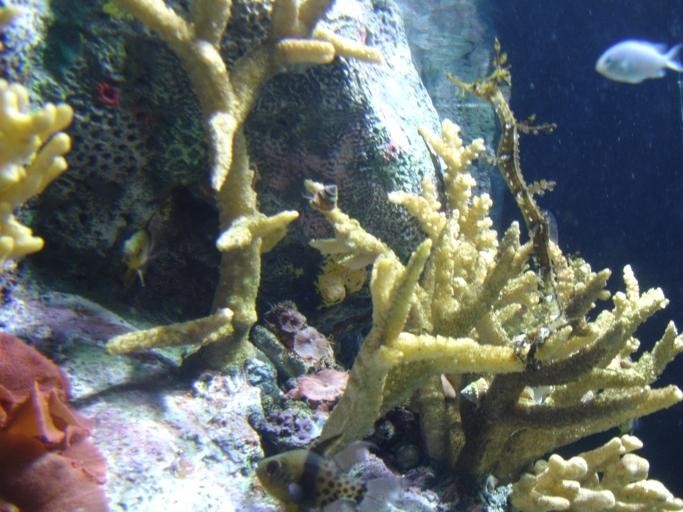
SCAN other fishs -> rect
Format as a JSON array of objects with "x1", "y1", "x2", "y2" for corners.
[
  {"x1": 254, "y1": 448, "x2": 368, "y2": 512},
  {"x1": 594, "y1": 37, "x2": 683, "y2": 85},
  {"x1": 121, "y1": 207, "x2": 158, "y2": 288}
]
